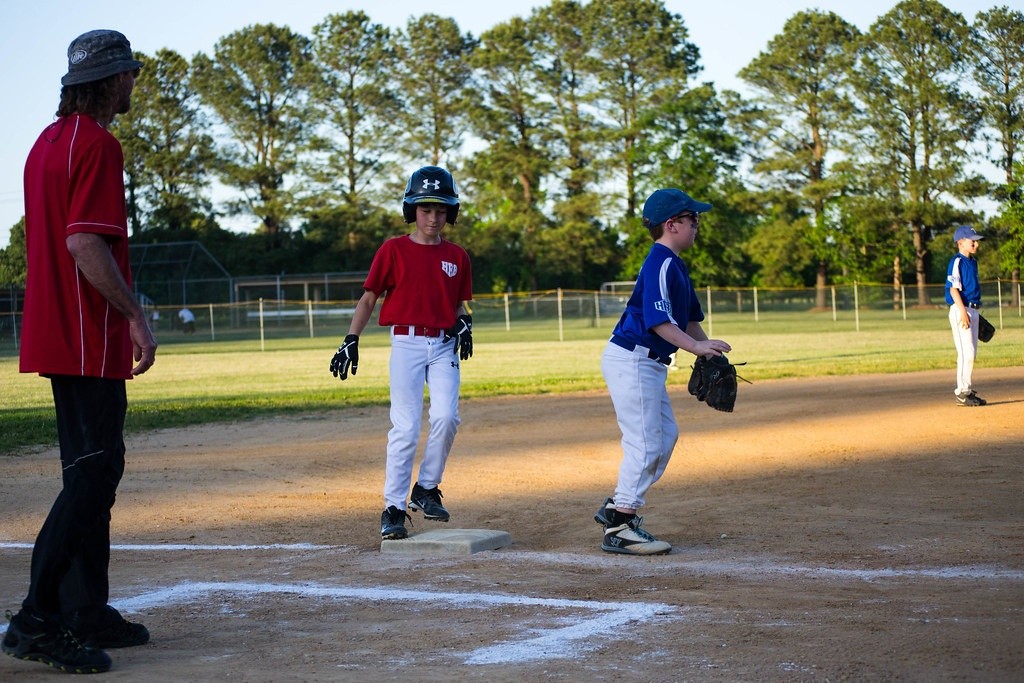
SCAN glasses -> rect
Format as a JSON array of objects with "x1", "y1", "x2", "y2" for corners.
[
  {"x1": 672, "y1": 211, "x2": 699, "y2": 224},
  {"x1": 132, "y1": 68, "x2": 139, "y2": 79}
]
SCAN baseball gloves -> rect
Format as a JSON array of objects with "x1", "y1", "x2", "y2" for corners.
[
  {"x1": 688, "y1": 350, "x2": 737, "y2": 413},
  {"x1": 978, "y1": 314, "x2": 995, "y2": 343}
]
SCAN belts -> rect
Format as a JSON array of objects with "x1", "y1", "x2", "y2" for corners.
[
  {"x1": 964, "y1": 302, "x2": 980, "y2": 309},
  {"x1": 610, "y1": 335, "x2": 672, "y2": 366},
  {"x1": 394, "y1": 325, "x2": 450, "y2": 337}
]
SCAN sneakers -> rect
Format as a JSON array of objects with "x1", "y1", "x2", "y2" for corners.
[
  {"x1": 956, "y1": 390, "x2": 986, "y2": 406},
  {"x1": 380, "y1": 506, "x2": 414, "y2": 539},
  {"x1": 595, "y1": 497, "x2": 616, "y2": 528},
  {"x1": 70, "y1": 606, "x2": 150, "y2": 649},
  {"x1": 601, "y1": 516, "x2": 673, "y2": 555},
  {"x1": 3, "y1": 609, "x2": 114, "y2": 674},
  {"x1": 408, "y1": 483, "x2": 450, "y2": 522}
]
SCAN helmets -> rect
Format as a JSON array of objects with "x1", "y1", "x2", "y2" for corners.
[{"x1": 402, "y1": 166, "x2": 459, "y2": 226}]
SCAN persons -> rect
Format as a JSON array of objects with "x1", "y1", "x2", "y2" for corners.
[
  {"x1": 149, "y1": 309, "x2": 159, "y2": 334},
  {"x1": 0, "y1": 29, "x2": 158, "y2": 673},
  {"x1": 177, "y1": 307, "x2": 198, "y2": 335},
  {"x1": 945, "y1": 224, "x2": 988, "y2": 406},
  {"x1": 592, "y1": 188, "x2": 732, "y2": 557},
  {"x1": 329, "y1": 166, "x2": 473, "y2": 540}
]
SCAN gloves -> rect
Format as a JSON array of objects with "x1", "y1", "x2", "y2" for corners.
[
  {"x1": 443, "y1": 315, "x2": 473, "y2": 360},
  {"x1": 329, "y1": 334, "x2": 358, "y2": 380}
]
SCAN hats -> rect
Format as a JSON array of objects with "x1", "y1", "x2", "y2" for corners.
[
  {"x1": 954, "y1": 225, "x2": 984, "y2": 242},
  {"x1": 643, "y1": 188, "x2": 713, "y2": 228},
  {"x1": 61, "y1": 29, "x2": 141, "y2": 86}
]
